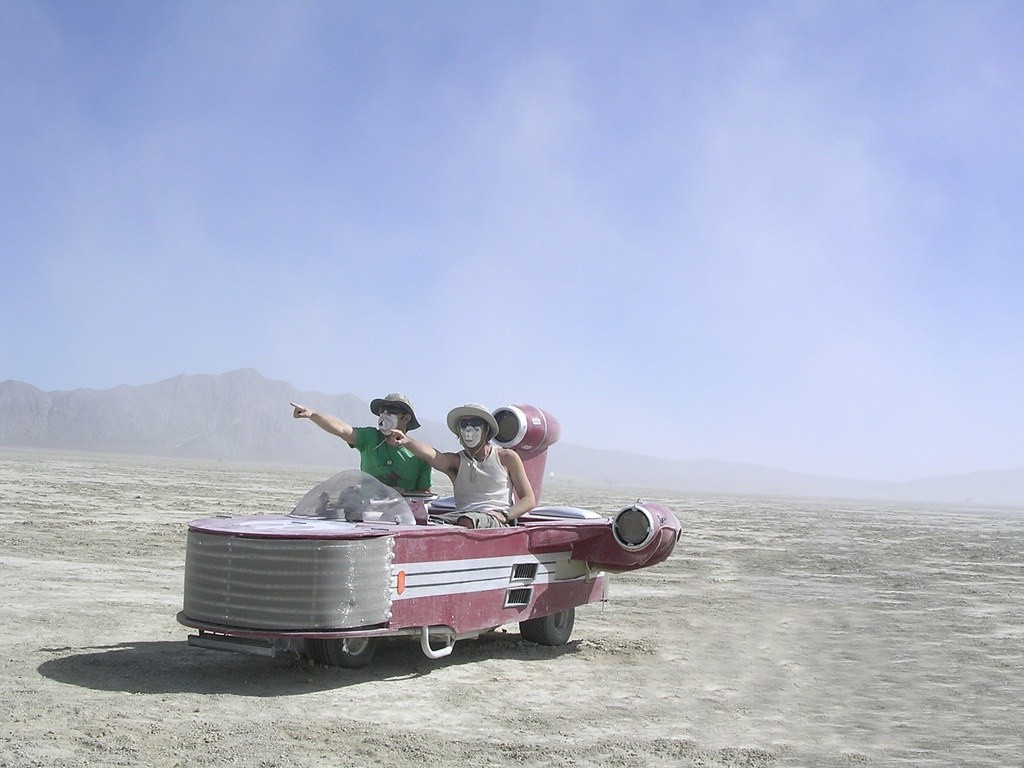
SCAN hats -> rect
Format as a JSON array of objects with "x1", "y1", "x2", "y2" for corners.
[
  {"x1": 447, "y1": 403, "x2": 499, "y2": 441},
  {"x1": 370, "y1": 393, "x2": 421, "y2": 431}
]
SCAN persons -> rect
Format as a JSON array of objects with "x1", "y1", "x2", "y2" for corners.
[
  {"x1": 289, "y1": 393, "x2": 432, "y2": 502},
  {"x1": 389, "y1": 402, "x2": 536, "y2": 530}
]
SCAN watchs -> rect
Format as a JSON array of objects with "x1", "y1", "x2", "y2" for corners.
[{"x1": 500, "y1": 509, "x2": 509, "y2": 524}]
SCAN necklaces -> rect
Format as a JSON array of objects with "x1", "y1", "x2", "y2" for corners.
[{"x1": 386, "y1": 443, "x2": 400, "y2": 465}]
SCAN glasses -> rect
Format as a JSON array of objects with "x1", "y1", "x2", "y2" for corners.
[
  {"x1": 378, "y1": 404, "x2": 407, "y2": 416},
  {"x1": 459, "y1": 418, "x2": 486, "y2": 430}
]
